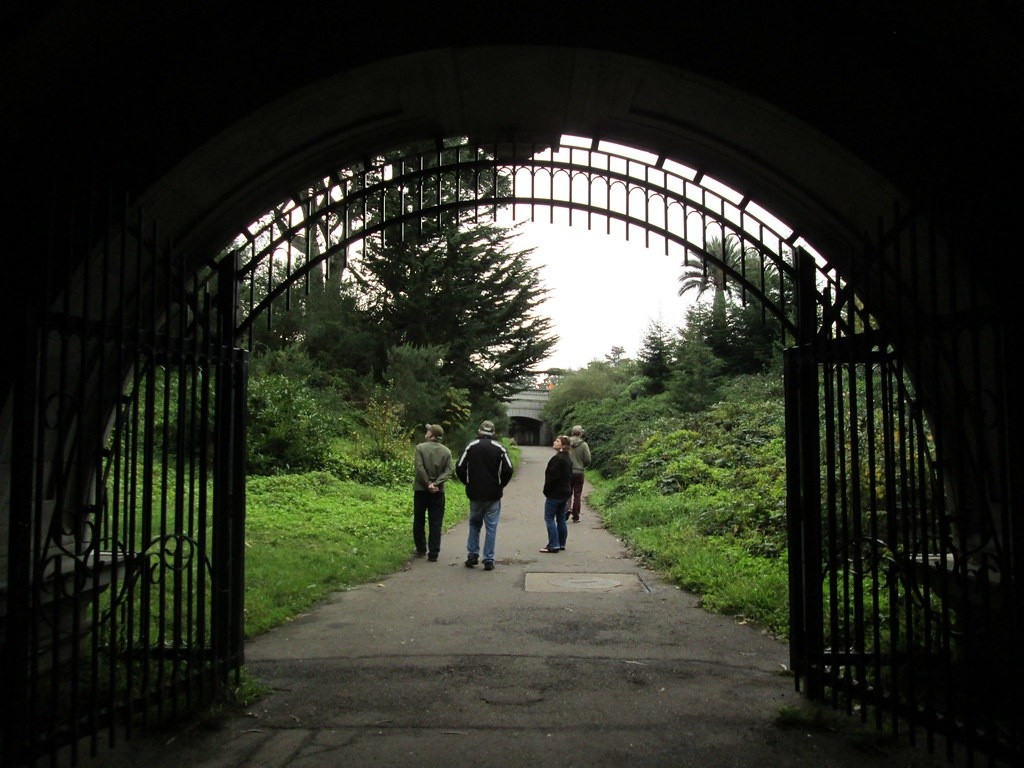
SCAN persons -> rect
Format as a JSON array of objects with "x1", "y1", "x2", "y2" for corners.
[
  {"x1": 539, "y1": 435, "x2": 573, "y2": 554},
  {"x1": 456, "y1": 421, "x2": 513, "y2": 570},
  {"x1": 566, "y1": 426, "x2": 591, "y2": 525},
  {"x1": 412, "y1": 424, "x2": 453, "y2": 562}
]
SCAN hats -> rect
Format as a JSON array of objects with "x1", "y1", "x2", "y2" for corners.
[
  {"x1": 425, "y1": 423, "x2": 444, "y2": 440},
  {"x1": 573, "y1": 425, "x2": 584, "y2": 435},
  {"x1": 478, "y1": 420, "x2": 495, "y2": 436}
]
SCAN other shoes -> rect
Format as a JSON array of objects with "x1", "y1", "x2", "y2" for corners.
[
  {"x1": 464, "y1": 557, "x2": 479, "y2": 565},
  {"x1": 558, "y1": 547, "x2": 565, "y2": 550},
  {"x1": 565, "y1": 510, "x2": 572, "y2": 521},
  {"x1": 538, "y1": 545, "x2": 559, "y2": 553},
  {"x1": 430, "y1": 558, "x2": 437, "y2": 561},
  {"x1": 574, "y1": 519, "x2": 584, "y2": 525},
  {"x1": 412, "y1": 547, "x2": 426, "y2": 556},
  {"x1": 484, "y1": 561, "x2": 496, "y2": 569}
]
